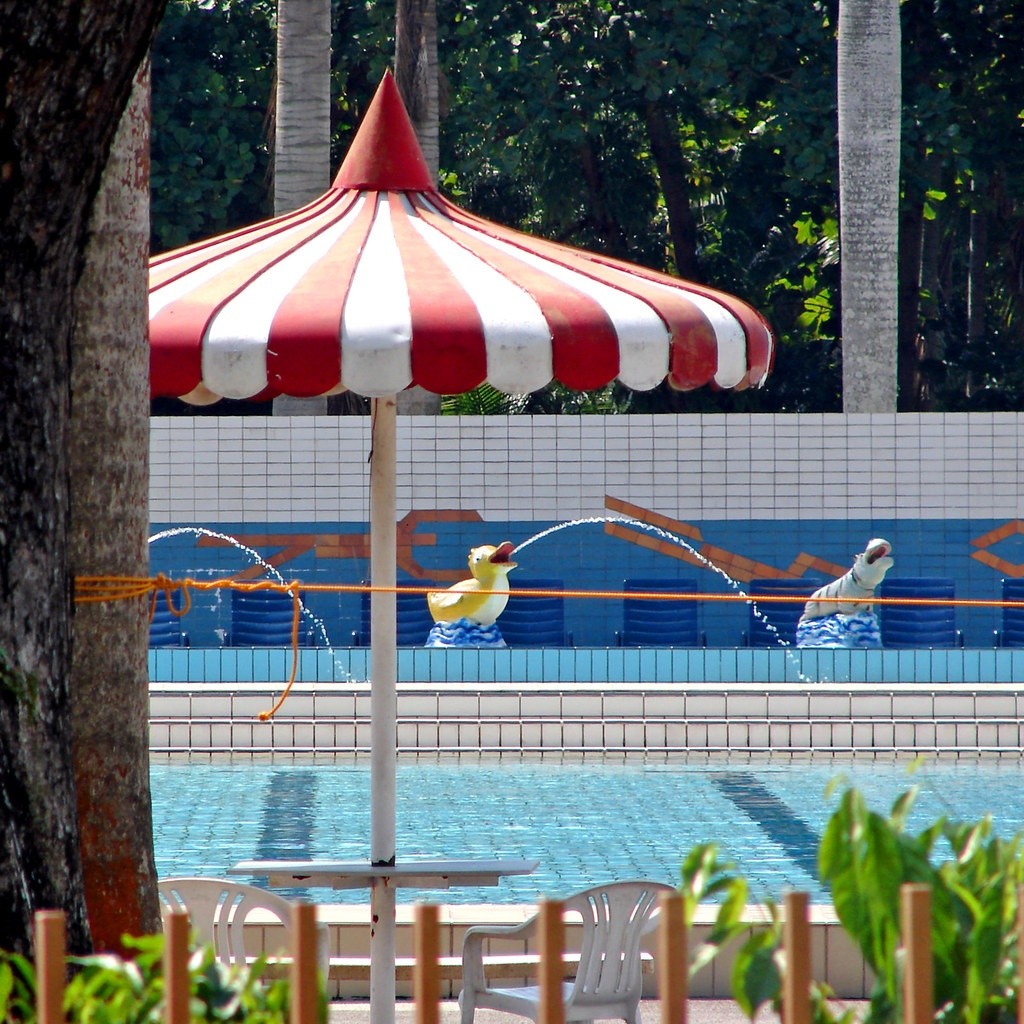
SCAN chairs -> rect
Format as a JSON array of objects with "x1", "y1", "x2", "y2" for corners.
[
  {"x1": 156, "y1": 879, "x2": 331, "y2": 995},
  {"x1": 457, "y1": 879, "x2": 679, "y2": 1024}
]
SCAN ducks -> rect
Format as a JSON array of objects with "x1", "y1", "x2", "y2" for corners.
[{"x1": 426, "y1": 540, "x2": 519, "y2": 624}]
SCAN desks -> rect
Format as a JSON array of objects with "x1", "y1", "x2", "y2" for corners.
[{"x1": 225, "y1": 859, "x2": 541, "y2": 1024}]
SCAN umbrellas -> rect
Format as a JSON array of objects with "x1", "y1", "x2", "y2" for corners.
[{"x1": 148, "y1": 66, "x2": 772, "y2": 1024}]
